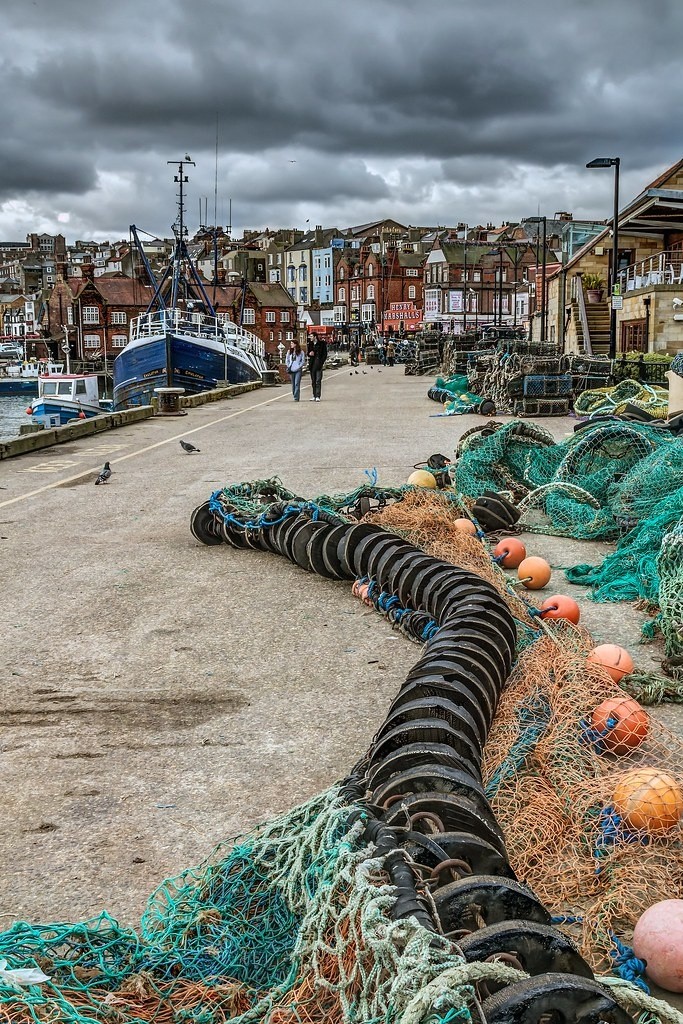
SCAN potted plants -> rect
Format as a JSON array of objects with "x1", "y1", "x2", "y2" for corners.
[{"x1": 582, "y1": 272, "x2": 605, "y2": 303}]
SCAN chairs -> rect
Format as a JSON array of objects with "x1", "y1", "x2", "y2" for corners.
[{"x1": 628, "y1": 264, "x2": 683, "y2": 291}]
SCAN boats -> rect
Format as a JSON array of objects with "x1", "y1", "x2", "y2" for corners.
[
  {"x1": 111, "y1": 160, "x2": 270, "y2": 411},
  {"x1": 26, "y1": 325, "x2": 115, "y2": 432},
  {"x1": 1, "y1": 356, "x2": 67, "y2": 395}
]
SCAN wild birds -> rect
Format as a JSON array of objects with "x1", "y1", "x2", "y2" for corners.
[{"x1": 349, "y1": 366, "x2": 383, "y2": 376}]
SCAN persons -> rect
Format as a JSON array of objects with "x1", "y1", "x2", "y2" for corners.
[
  {"x1": 285, "y1": 339, "x2": 305, "y2": 402},
  {"x1": 306, "y1": 331, "x2": 327, "y2": 401},
  {"x1": 383, "y1": 340, "x2": 395, "y2": 366},
  {"x1": 381, "y1": 338, "x2": 388, "y2": 366}
]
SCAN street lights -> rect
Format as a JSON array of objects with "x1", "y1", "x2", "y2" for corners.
[
  {"x1": 585, "y1": 155, "x2": 623, "y2": 377},
  {"x1": 523, "y1": 216, "x2": 549, "y2": 341},
  {"x1": 485, "y1": 250, "x2": 504, "y2": 326}
]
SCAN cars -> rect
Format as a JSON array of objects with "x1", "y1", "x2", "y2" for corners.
[{"x1": 0, "y1": 341, "x2": 24, "y2": 358}]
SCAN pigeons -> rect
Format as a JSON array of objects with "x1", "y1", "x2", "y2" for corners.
[
  {"x1": 94, "y1": 462, "x2": 112, "y2": 486},
  {"x1": 179, "y1": 440, "x2": 201, "y2": 455}
]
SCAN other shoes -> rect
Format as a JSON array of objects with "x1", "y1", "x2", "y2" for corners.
[
  {"x1": 310, "y1": 397, "x2": 320, "y2": 402},
  {"x1": 295, "y1": 398, "x2": 299, "y2": 401}
]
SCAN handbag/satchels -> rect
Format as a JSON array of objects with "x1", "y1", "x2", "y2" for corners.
[{"x1": 286, "y1": 367, "x2": 291, "y2": 374}]
